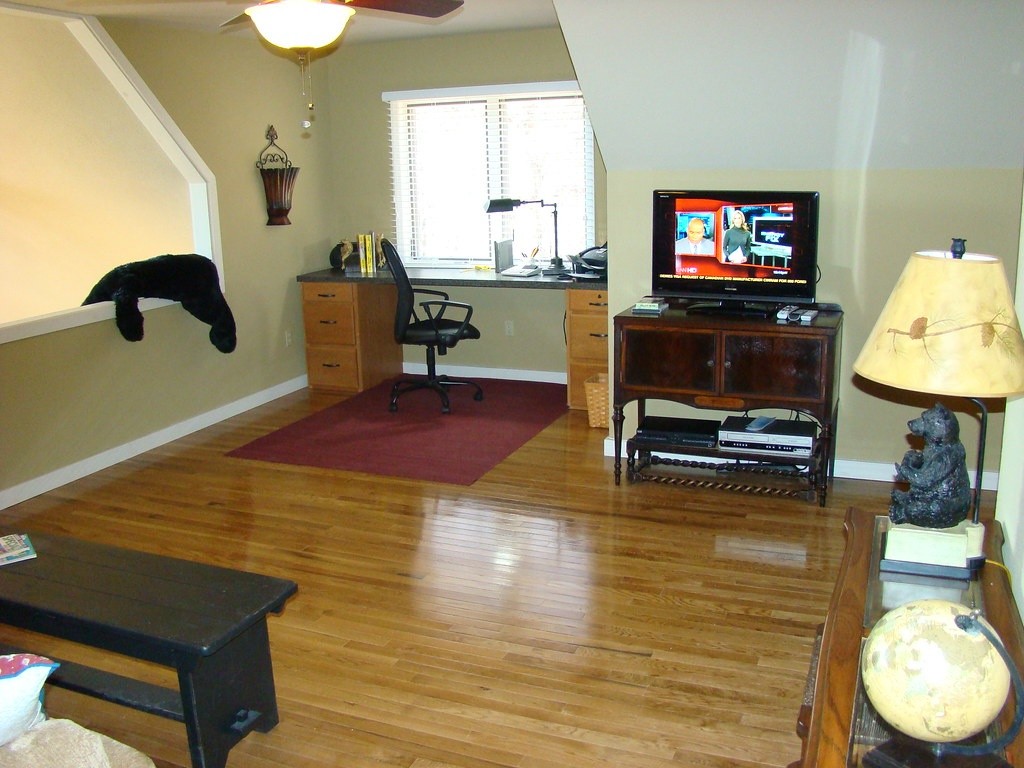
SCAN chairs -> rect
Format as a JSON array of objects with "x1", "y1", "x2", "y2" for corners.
[{"x1": 381, "y1": 239, "x2": 482, "y2": 414}]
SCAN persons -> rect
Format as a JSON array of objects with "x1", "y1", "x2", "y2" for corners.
[
  {"x1": 675, "y1": 218, "x2": 714, "y2": 255},
  {"x1": 723, "y1": 210, "x2": 751, "y2": 263}
]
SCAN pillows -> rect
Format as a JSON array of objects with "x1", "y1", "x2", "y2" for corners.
[{"x1": 0, "y1": 654, "x2": 61, "y2": 746}]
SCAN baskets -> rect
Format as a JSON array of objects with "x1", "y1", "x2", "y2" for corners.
[{"x1": 583, "y1": 373, "x2": 611, "y2": 428}]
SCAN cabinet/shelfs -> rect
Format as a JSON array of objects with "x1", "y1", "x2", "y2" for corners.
[
  {"x1": 791, "y1": 498, "x2": 1024, "y2": 768},
  {"x1": 301, "y1": 280, "x2": 403, "y2": 394},
  {"x1": 610, "y1": 297, "x2": 846, "y2": 505},
  {"x1": 565, "y1": 288, "x2": 607, "y2": 410}
]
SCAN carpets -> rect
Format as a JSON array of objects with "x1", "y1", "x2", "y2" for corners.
[{"x1": 223, "y1": 372, "x2": 569, "y2": 487}]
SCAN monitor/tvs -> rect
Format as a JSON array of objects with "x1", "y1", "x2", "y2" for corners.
[{"x1": 651, "y1": 190, "x2": 819, "y2": 317}]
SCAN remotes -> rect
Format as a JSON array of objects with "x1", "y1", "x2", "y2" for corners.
[{"x1": 776, "y1": 305, "x2": 819, "y2": 322}]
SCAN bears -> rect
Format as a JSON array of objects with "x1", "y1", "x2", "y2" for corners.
[{"x1": 889, "y1": 402, "x2": 972, "y2": 528}]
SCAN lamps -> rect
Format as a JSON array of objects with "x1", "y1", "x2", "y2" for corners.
[
  {"x1": 486, "y1": 198, "x2": 571, "y2": 276},
  {"x1": 256, "y1": 126, "x2": 301, "y2": 225},
  {"x1": 851, "y1": 235, "x2": 1024, "y2": 568},
  {"x1": 243, "y1": 2, "x2": 356, "y2": 130}
]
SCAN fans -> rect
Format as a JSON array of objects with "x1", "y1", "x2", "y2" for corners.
[{"x1": 220, "y1": 0, "x2": 463, "y2": 19}]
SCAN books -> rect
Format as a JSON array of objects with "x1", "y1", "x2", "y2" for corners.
[
  {"x1": 0, "y1": 533, "x2": 37, "y2": 565},
  {"x1": 633, "y1": 298, "x2": 669, "y2": 313},
  {"x1": 357, "y1": 234, "x2": 373, "y2": 273}
]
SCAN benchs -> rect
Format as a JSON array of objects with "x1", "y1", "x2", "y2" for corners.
[{"x1": 0, "y1": 518, "x2": 298, "y2": 768}]
